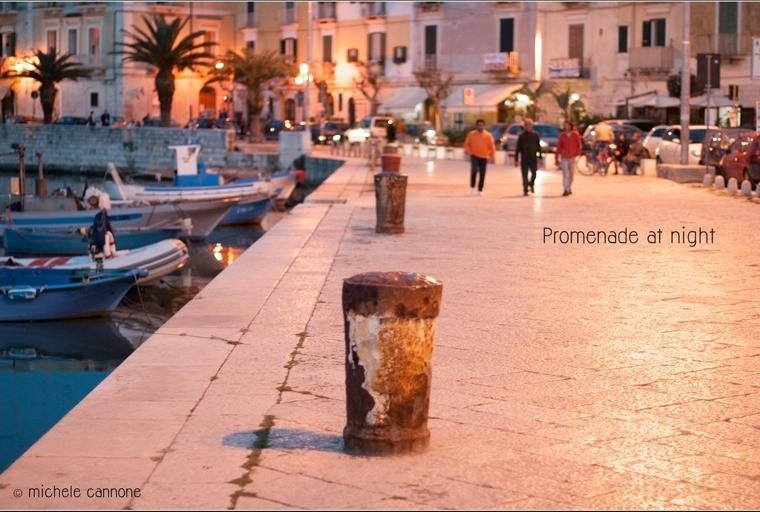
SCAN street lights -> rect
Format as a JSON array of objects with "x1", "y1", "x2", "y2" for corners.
[{"x1": 294, "y1": 62, "x2": 314, "y2": 147}]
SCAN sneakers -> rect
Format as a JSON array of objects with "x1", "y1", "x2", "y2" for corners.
[
  {"x1": 563, "y1": 189, "x2": 572, "y2": 196},
  {"x1": 524, "y1": 182, "x2": 534, "y2": 196},
  {"x1": 471, "y1": 188, "x2": 483, "y2": 196}
]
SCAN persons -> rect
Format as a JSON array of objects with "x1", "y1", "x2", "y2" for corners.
[
  {"x1": 513, "y1": 119, "x2": 543, "y2": 195},
  {"x1": 397, "y1": 118, "x2": 408, "y2": 144},
  {"x1": 581, "y1": 120, "x2": 616, "y2": 168},
  {"x1": 609, "y1": 129, "x2": 629, "y2": 175},
  {"x1": 624, "y1": 130, "x2": 644, "y2": 175},
  {"x1": 386, "y1": 120, "x2": 396, "y2": 142},
  {"x1": 87, "y1": 110, "x2": 96, "y2": 127},
  {"x1": 462, "y1": 119, "x2": 496, "y2": 194},
  {"x1": 100, "y1": 110, "x2": 111, "y2": 127},
  {"x1": 555, "y1": 118, "x2": 582, "y2": 197}
]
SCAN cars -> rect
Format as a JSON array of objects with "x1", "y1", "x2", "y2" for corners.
[{"x1": 486, "y1": 119, "x2": 760, "y2": 191}]
